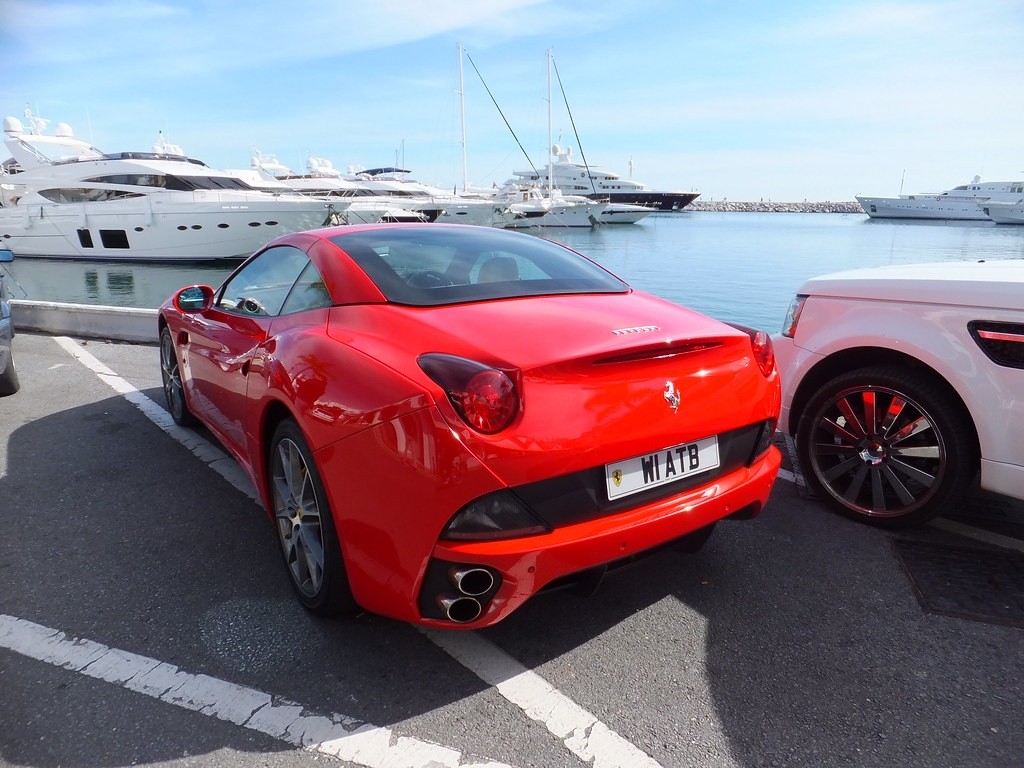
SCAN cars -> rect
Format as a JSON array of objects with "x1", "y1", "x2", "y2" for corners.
[
  {"x1": 0, "y1": 249, "x2": 20, "y2": 396},
  {"x1": 769, "y1": 257, "x2": 1024, "y2": 529}
]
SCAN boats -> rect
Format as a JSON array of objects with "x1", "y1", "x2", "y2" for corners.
[
  {"x1": 853, "y1": 181, "x2": 1024, "y2": 221},
  {"x1": 0, "y1": 43, "x2": 702, "y2": 259},
  {"x1": 976, "y1": 198, "x2": 1023, "y2": 224}
]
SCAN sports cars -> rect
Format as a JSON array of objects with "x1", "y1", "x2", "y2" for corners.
[{"x1": 157, "y1": 221, "x2": 782, "y2": 632}]
